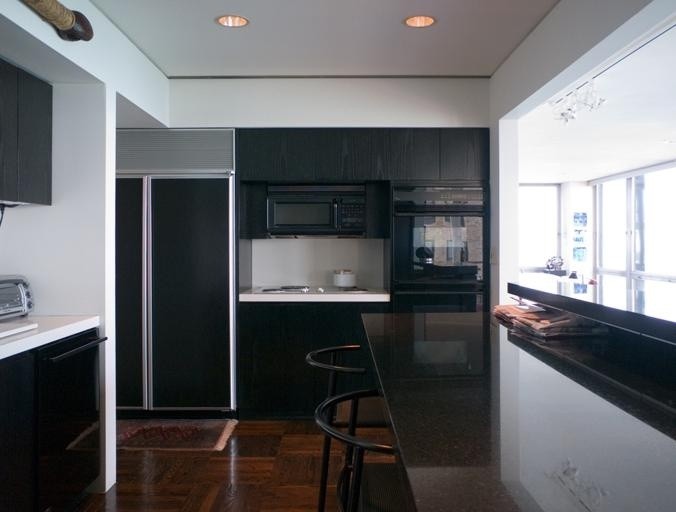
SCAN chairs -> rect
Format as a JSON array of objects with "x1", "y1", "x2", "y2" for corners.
[
  {"x1": 315, "y1": 388, "x2": 409, "y2": 511},
  {"x1": 308, "y1": 342, "x2": 375, "y2": 398}
]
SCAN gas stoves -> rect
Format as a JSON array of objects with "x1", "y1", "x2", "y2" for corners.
[{"x1": 255, "y1": 283, "x2": 375, "y2": 294}]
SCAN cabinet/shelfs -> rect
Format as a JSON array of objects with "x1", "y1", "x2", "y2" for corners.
[
  {"x1": 236, "y1": 285, "x2": 391, "y2": 418},
  {"x1": 115, "y1": 126, "x2": 234, "y2": 415},
  {"x1": 0, "y1": 313, "x2": 107, "y2": 512},
  {"x1": 233, "y1": 127, "x2": 489, "y2": 184}
]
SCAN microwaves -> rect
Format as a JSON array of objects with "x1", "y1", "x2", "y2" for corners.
[{"x1": 266, "y1": 193, "x2": 368, "y2": 237}]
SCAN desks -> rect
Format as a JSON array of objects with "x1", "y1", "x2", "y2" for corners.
[{"x1": 361, "y1": 308, "x2": 676, "y2": 512}]
